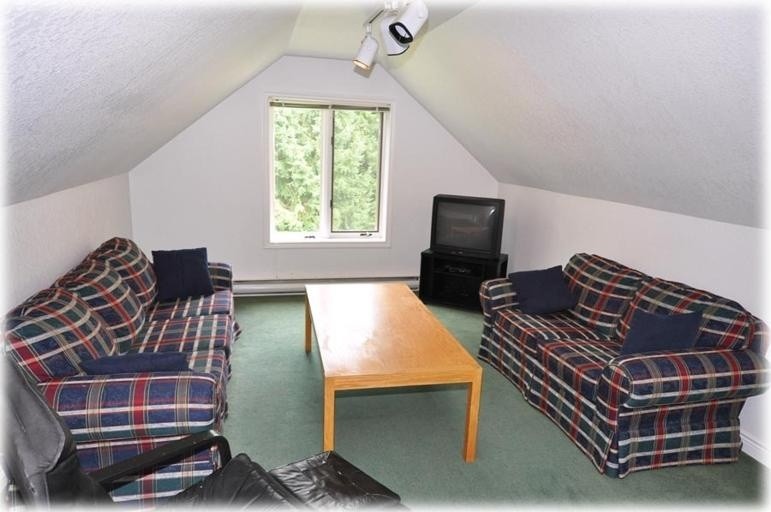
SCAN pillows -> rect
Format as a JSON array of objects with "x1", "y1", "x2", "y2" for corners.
[
  {"x1": 508, "y1": 252, "x2": 755, "y2": 356},
  {"x1": 0, "y1": 238, "x2": 215, "y2": 384}
]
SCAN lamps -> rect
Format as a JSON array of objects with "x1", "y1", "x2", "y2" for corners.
[{"x1": 353, "y1": 0, "x2": 430, "y2": 70}]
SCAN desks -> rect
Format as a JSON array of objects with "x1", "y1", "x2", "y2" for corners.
[{"x1": 306, "y1": 283, "x2": 485, "y2": 463}]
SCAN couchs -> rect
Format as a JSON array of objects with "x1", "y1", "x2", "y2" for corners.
[
  {"x1": 476, "y1": 254, "x2": 767, "y2": 480},
  {"x1": 0, "y1": 238, "x2": 241, "y2": 512}
]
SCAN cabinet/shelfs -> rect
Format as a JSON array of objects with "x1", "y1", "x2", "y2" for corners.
[{"x1": 419, "y1": 248, "x2": 508, "y2": 312}]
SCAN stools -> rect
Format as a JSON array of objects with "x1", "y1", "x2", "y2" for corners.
[{"x1": 270, "y1": 451, "x2": 400, "y2": 511}]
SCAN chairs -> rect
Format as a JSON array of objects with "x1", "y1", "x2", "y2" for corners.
[{"x1": 1, "y1": 355, "x2": 311, "y2": 512}]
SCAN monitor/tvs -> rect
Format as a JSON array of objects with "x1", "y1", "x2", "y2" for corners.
[{"x1": 430, "y1": 195, "x2": 504, "y2": 260}]
